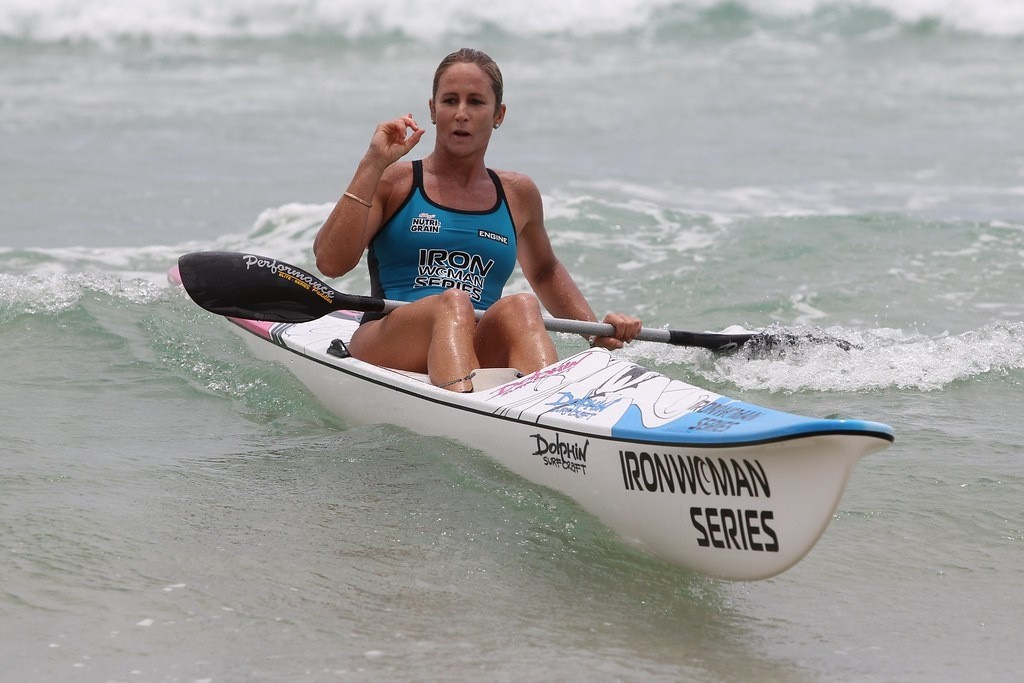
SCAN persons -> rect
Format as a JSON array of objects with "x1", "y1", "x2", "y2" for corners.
[{"x1": 314, "y1": 48, "x2": 642, "y2": 395}]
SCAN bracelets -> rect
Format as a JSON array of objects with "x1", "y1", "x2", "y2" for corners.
[
  {"x1": 344, "y1": 191, "x2": 372, "y2": 208},
  {"x1": 589, "y1": 336, "x2": 595, "y2": 344}
]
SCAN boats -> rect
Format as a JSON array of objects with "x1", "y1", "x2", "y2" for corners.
[{"x1": 167, "y1": 264, "x2": 896, "y2": 580}]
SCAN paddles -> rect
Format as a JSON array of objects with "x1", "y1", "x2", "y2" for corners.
[{"x1": 175, "y1": 250, "x2": 860, "y2": 356}]
